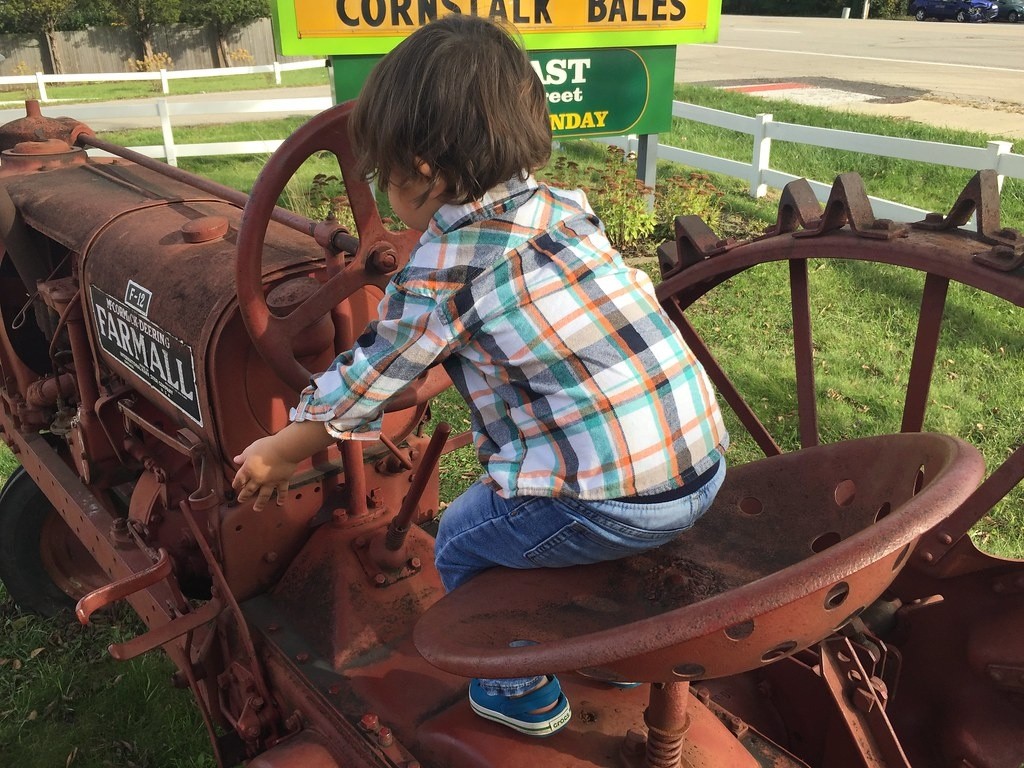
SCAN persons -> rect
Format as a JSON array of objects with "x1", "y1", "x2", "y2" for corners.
[{"x1": 234, "y1": 15, "x2": 729, "y2": 734}]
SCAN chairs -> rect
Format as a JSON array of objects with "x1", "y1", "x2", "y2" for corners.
[{"x1": 412, "y1": 431, "x2": 985, "y2": 684}]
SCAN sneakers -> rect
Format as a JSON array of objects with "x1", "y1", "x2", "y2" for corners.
[
  {"x1": 577, "y1": 666, "x2": 643, "y2": 689},
  {"x1": 469, "y1": 674, "x2": 573, "y2": 738}
]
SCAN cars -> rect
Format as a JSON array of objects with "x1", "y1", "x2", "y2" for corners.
[{"x1": 908, "y1": 0, "x2": 1024, "y2": 23}]
